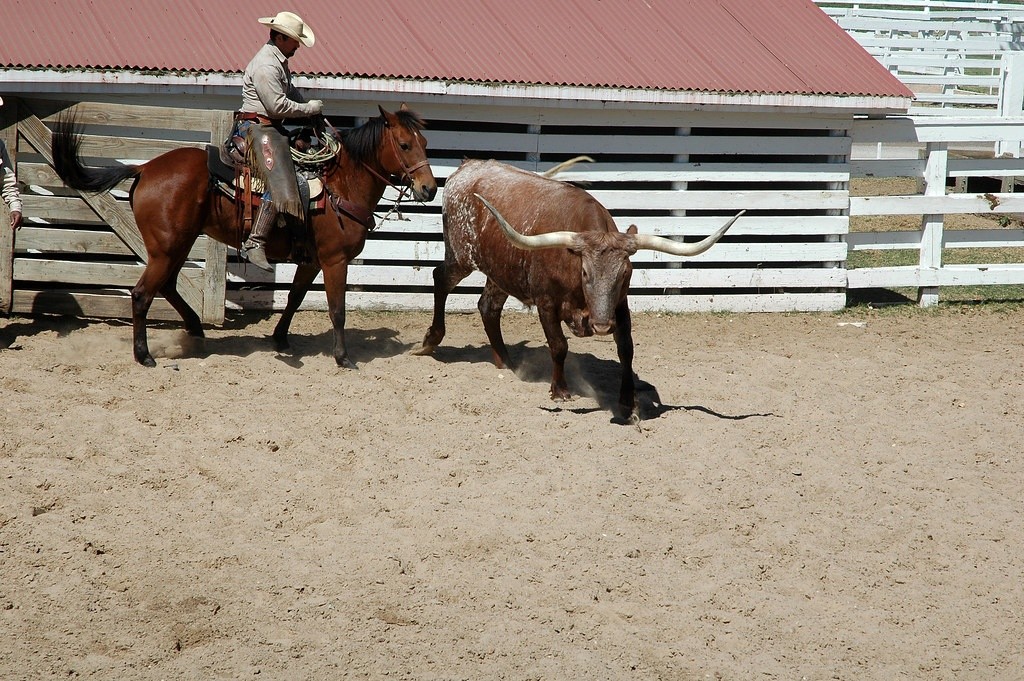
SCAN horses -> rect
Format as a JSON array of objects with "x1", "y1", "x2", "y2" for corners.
[{"x1": 51, "y1": 100, "x2": 438, "y2": 370}]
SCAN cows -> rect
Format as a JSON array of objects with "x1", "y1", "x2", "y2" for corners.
[{"x1": 409, "y1": 156, "x2": 746, "y2": 421}]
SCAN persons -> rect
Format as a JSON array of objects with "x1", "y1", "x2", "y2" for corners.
[{"x1": 237, "y1": 11, "x2": 324, "y2": 273}]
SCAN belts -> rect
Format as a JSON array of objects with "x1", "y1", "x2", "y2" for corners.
[{"x1": 235, "y1": 113, "x2": 273, "y2": 125}]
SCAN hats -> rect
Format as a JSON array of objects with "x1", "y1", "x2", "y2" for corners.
[{"x1": 258, "y1": 12, "x2": 315, "y2": 48}]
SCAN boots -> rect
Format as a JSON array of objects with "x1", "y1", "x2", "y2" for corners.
[{"x1": 240, "y1": 199, "x2": 278, "y2": 273}]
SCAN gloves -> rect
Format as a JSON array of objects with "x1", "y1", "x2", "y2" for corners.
[{"x1": 308, "y1": 100, "x2": 323, "y2": 115}]
